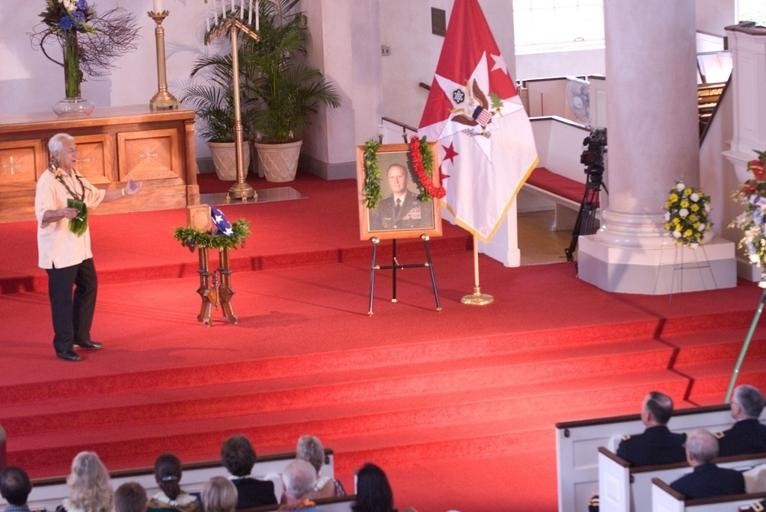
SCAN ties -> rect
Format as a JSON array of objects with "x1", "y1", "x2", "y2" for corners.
[{"x1": 394, "y1": 198, "x2": 402, "y2": 217}]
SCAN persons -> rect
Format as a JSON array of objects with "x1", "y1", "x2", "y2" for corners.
[
  {"x1": 149, "y1": 454, "x2": 202, "y2": 512},
  {"x1": 712, "y1": 384, "x2": 766, "y2": 457},
  {"x1": 279, "y1": 460, "x2": 321, "y2": 511},
  {"x1": 349, "y1": 463, "x2": 398, "y2": 512},
  {"x1": 218, "y1": 435, "x2": 277, "y2": 510},
  {"x1": 34, "y1": 133, "x2": 144, "y2": 361},
  {"x1": 668, "y1": 427, "x2": 746, "y2": 501},
  {"x1": 280, "y1": 435, "x2": 346, "y2": 503},
  {"x1": 201, "y1": 476, "x2": 238, "y2": 512},
  {"x1": 588, "y1": 392, "x2": 686, "y2": 511},
  {"x1": 110, "y1": 481, "x2": 148, "y2": 512},
  {"x1": 55, "y1": 452, "x2": 112, "y2": 512},
  {"x1": 1, "y1": 466, "x2": 34, "y2": 512},
  {"x1": 369, "y1": 163, "x2": 433, "y2": 230}
]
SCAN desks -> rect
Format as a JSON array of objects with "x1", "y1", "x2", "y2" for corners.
[{"x1": 0, "y1": 105, "x2": 201, "y2": 224}]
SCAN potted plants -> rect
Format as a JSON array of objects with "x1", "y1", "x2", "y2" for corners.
[
  {"x1": 229, "y1": 0, "x2": 343, "y2": 182},
  {"x1": 179, "y1": 8, "x2": 295, "y2": 181}
]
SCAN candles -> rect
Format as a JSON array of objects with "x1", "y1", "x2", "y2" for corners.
[
  {"x1": 222, "y1": 0, "x2": 226, "y2": 18},
  {"x1": 206, "y1": 17, "x2": 210, "y2": 32},
  {"x1": 214, "y1": 12, "x2": 218, "y2": 26},
  {"x1": 153, "y1": 0, "x2": 164, "y2": 11},
  {"x1": 231, "y1": 0, "x2": 235, "y2": 13},
  {"x1": 248, "y1": 0, "x2": 253, "y2": 24},
  {"x1": 255, "y1": 0, "x2": 259, "y2": 32},
  {"x1": 239, "y1": 0, "x2": 245, "y2": 20}
]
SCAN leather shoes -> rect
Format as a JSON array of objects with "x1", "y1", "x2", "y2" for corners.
[
  {"x1": 58, "y1": 351, "x2": 80, "y2": 361},
  {"x1": 73, "y1": 339, "x2": 103, "y2": 350}
]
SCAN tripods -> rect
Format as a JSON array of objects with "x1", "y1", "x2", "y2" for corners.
[{"x1": 564, "y1": 170, "x2": 609, "y2": 262}]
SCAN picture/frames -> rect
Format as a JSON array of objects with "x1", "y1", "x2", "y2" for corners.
[{"x1": 356, "y1": 141, "x2": 442, "y2": 241}]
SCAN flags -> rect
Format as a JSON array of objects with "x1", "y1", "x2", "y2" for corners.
[{"x1": 414, "y1": 1, "x2": 539, "y2": 243}]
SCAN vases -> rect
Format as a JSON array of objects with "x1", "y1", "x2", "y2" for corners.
[{"x1": 52, "y1": 98, "x2": 95, "y2": 117}]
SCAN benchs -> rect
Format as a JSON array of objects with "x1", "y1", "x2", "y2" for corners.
[{"x1": 521, "y1": 167, "x2": 600, "y2": 231}]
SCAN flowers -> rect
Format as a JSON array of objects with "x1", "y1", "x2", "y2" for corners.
[
  {"x1": 733, "y1": 158, "x2": 766, "y2": 303},
  {"x1": 38, "y1": 0, "x2": 101, "y2": 99},
  {"x1": 410, "y1": 137, "x2": 445, "y2": 201},
  {"x1": 665, "y1": 181, "x2": 710, "y2": 248}
]
